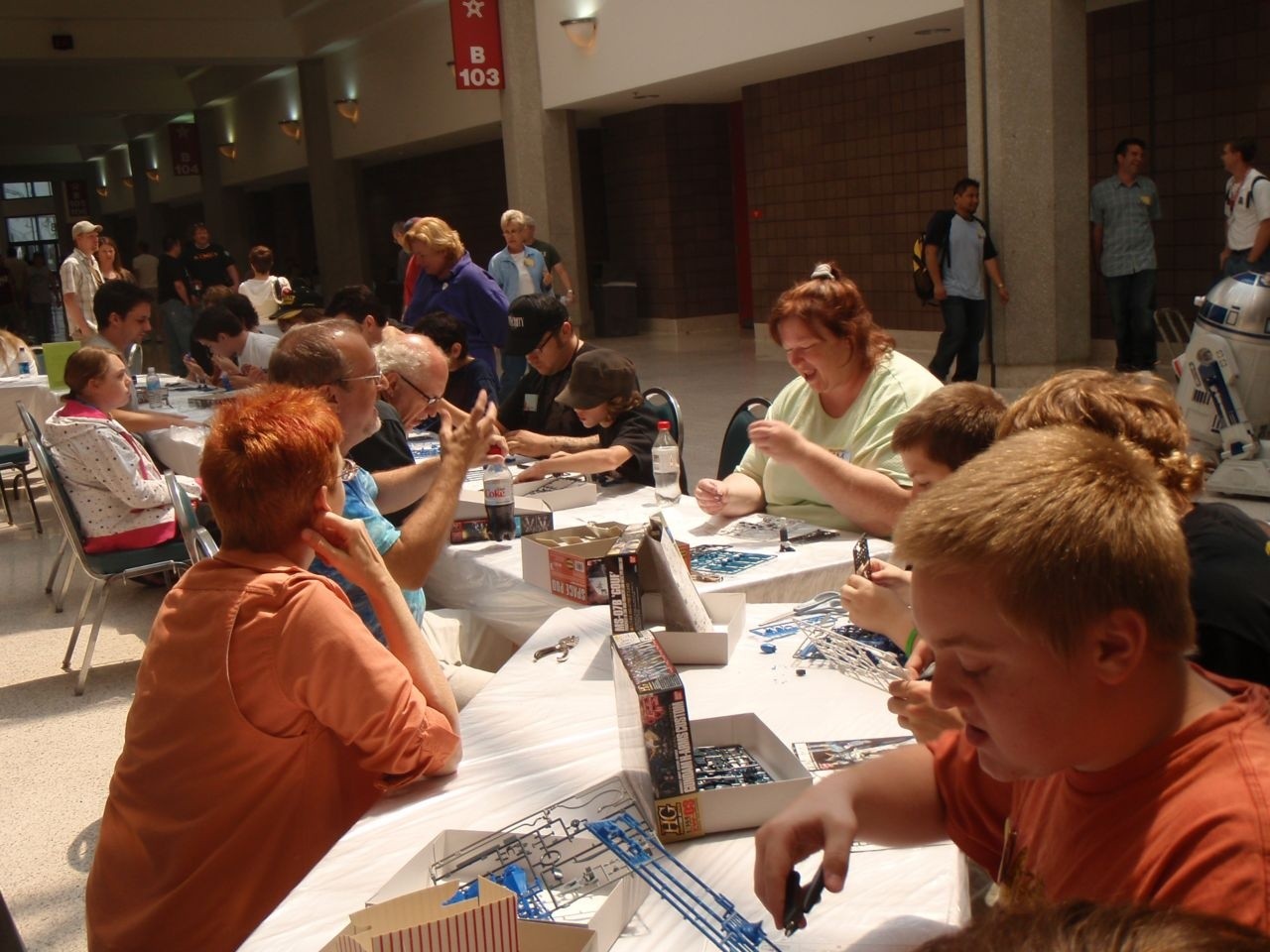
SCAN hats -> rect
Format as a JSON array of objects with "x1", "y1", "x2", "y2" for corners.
[
  {"x1": 72, "y1": 221, "x2": 102, "y2": 239},
  {"x1": 269, "y1": 286, "x2": 325, "y2": 321},
  {"x1": 495, "y1": 294, "x2": 569, "y2": 354},
  {"x1": 554, "y1": 348, "x2": 636, "y2": 410}
]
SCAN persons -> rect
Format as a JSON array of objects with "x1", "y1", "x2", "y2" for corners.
[
  {"x1": 71, "y1": 281, "x2": 214, "y2": 436},
  {"x1": 34, "y1": 341, "x2": 223, "y2": 580},
  {"x1": 1088, "y1": 137, "x2": 1165, "y2": 375},
  {"x1": 925, "y1": 177, "x2": 1012, "y2": 389},
  {"x1": 1211, "y1": 133, "x2": 1270, "y2": 288},
  {"x1": 503, "y1": 340, "x2": 689, "y2": 496},
  {"x1": 1, "y1": 209, "x2": 1270, "y2": 951},
  {"x1": 695, "y1": 256, "x2": 963, "y2": 540}
]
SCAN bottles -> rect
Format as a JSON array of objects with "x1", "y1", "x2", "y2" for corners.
[
  {"x1": 18, "y1": 347, "x2": 32, "y2": 382},
  {"x1": 146, "y1": 366, "x2": 163, "y2": 409},
  {"x1": 483, "y1": 444, "x2": 517, "y2": 541},
  {"x1": 651, "y1": 421, "x2": 682, "y2": 507}
]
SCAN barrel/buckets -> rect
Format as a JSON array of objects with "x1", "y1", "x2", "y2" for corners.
[{"x1": 42, "y1": 341, "x2": 82, "y2": 390}]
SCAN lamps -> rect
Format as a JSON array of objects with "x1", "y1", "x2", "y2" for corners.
[
  {"x1": 559, "y1": 17, "x2": 597, "y2": 48},
  {"x1": 333, "y1": 99, "x2": 357, "y2": 119},
  {"x1": 278, "y1": 120, "x2": 300, "y2": 138},
  {"x1": 217, "y1": 142, "x2": 235, "y2": 158}
]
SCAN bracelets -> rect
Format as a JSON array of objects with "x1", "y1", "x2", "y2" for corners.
[
  {"x1": 997, "y1": 283, "x2": 1005, "y2": 289},
  {"x1": 566, "y1": 288, "x2": 574, "y2": 294}
]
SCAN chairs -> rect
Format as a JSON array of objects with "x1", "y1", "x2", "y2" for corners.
[
  {"x1": 16, "y1": 400, "x2": 77, "y2": 613},
  {"x1": 164, "y1": 470, "x2": 220, "y2": 564},
  {"x1": 26, "y1": 431, "x2": 191, "y2": 697},
  {"x1": 716, "y1": 396, "x2": 773, "y2": 480},
  {"x1": 642, "y1": 386, "x2": 684, "y2": 459}
]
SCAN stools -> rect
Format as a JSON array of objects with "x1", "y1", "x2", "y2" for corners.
[{"x1": 0, "y1": 445, "x2": 43, "y2": 534}]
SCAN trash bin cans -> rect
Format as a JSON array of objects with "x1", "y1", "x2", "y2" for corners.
[{"x1": 602, "y1": 282, "x2": 637, "y2": 336}]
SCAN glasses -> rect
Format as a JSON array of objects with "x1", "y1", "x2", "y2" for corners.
[
  {"x1": 331, "y1": 458, "x2": 360, "y2": 482},
  {"x1": 393, "y1": 373, "x2": 443, "y2": 405},
  {"x1": 531, "y1": 331, "x2": 555, "y2": 355},
  {"x1": 338, "y1": 365, "x2": 382, "y2": 386}
]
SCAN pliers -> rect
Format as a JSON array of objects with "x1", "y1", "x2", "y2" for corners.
[
  {"x1": 534, "y1": 636, "x2": 575, "y2": 662},
  {"x1": 690, "y1": 571, "x2": 719, "y2": 582},
  {"x1": 784, "y1": 862, "x2": 825, "y2": 936}
]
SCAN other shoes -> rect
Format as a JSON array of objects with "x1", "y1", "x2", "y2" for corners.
[{"x1": 134, "y1": 572, "x2": 177, "y2": 588}]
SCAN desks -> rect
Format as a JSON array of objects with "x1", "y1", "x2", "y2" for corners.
[{"x1": 0, "y1": 298, "x2": 971, "y2": 952}]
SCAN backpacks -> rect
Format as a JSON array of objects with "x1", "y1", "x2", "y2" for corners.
[{"x1": 913, "y1": 210, "x2": 950, "y2": 304}]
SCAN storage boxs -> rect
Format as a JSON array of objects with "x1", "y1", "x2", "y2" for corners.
[
  {"x1": 605, "y1": 522, "x2": 745, "y2": 665},
  {"x1": 449, "y1": 490, "x2": 553, "y2": 545},
  {"x1": 318, "y1": 911, "x2": 598, "y2": 952},
  {"x1": 334, "y1": 870, "x2": 520, "y2": 952},
  {"x1": 523, "y1": 519, "x2": 624, "y2": 593},
  {"x1": 609, "y1": 630, "x2": 813, "y2": 844},
  {"x1": 549, "y1": 534, "x2": 691, "y2": 606},
  {"x1": 365, "y1": 829, "x2": 652, "y2": 952},
  {"x1": 513, "y1": 478, "x2": 596, "y2": 511}
]
